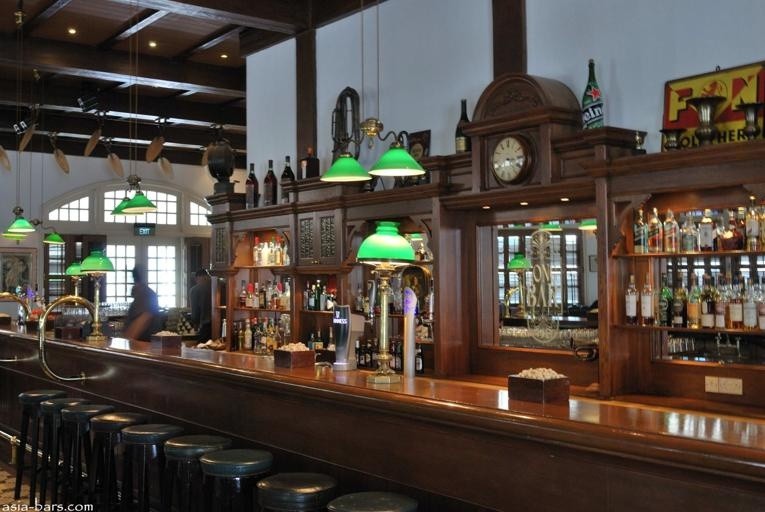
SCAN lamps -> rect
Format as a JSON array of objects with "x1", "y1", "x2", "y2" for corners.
[
  {"x1": 1, "y1": 219, "x2": 65, "y2": 245},
  {"x1": 320, "y1": 118, "x2": 426, "y2": 182},
  {"x1": 1, "y1": 206, "x2": 36, "y2": 237},
  {"x1": 356, "y1": 226, "x2": 417, "y2": 383},
  {"x1": 539, "y1": 224, "x2": 562, "y2": 231},
  {"x1": 80, "y1": 251, "x2": 116, "y2": 341},
  {"x1": 507, "y1": 255, "x2": 533, "y2": 318},
  {"x1": 111, "y1": 175, "x2": 158, "y2": 215},
  {"x1": 577, "y1": 217, "x2": 598, "y2": 231},
  {"x1": 66, "y1": 263, "x2": 88, "y2": 306}
]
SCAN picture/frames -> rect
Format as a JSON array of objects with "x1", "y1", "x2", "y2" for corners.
[{"x1": 0, "y1": 247, "x2": 37, "y2": 302}]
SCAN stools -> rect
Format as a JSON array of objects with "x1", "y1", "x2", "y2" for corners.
[
  {"x1": 199, "y1": 449, "x2": 273, "y2": 512},
  {"x1": 256, "y1": 472, "x2": 337, "y2": 512},
  {"x1": 14, "y1": 389, "x2": 68, "y2": 509},
  {"x1": 161, "y1": 435, "x2": 223, "y2": 512},
  {"x1": 327, "y1": 491, "x2": 419, "y2": 512},
  {"x1": 39, "y1": 398, "x2": 91, "y2": 512},
  {"x1": 87, "y1": 411, "x2": 145, "y2": 512},
  {"x1": 120, "y1": 424, "x2": 175, "y2": 512},
  {"x1": 61, "y1": 404, "x2": 115, "y2": 512}
]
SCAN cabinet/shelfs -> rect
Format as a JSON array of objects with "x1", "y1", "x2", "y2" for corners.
[
  {"x1": 231, "y1": 214, "x2": 292, "y2": 355},
  {"x1": 343, "y1": 211, "x2": 437, "y2": 379},
  {"x1": 206, "y1": 222, "x2": 229, "y2": 351},
  {"x1": 599, "y1": 138, "x2": 765, "y2": 421},
  {"x1": 292, "y1": 210, "x2": 340, "y2": 366}
]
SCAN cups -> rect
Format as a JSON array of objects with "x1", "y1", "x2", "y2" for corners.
[
  {"x1": 61, "y1": 301, "x2": 130, "y2": 324},
  {"x1": 494, "y1": 326, "x2": 599, "y2": 350}
]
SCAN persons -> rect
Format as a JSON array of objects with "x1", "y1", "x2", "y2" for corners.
[
  {"x1": 121, "y1": 264, "x2": 159, "y2": 345},
  {"x1": 190, "y1": 269, "x2": 225, "y2": 342}
]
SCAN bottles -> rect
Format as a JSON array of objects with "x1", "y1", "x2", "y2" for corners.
[
  {"x1": 663, "y1": 209, "x2": 680, "y2": 253},
  {"x1": 700, "y1": 209, "x2": 714, "y2": 251},
  {"x1": 230, "y1": 320, "x2": 239, "y2": 351},
  {"x1": 282, "y1": 244, "x2": 290, "y2": 266},
  {"x1": 284, "y1": 319, "x2": 291, "y2": 346},
  {"x1": 355, "y1": 289, "x2": 366, "y2": 312},
  {"x1": 319, "y1": 286, "x2": 327, "y2": 311},
  {"x1": 254, "y1": 324, "x2": 262, "y2": 354},
  {"x1": 699, "y1": 274, "x2": 708, "y2": 301},
  {"x1": 252, "y1": 283, "x2": 260, "y2": 309},
  {"x1": 702, "y1": 276, "x2": 716, "y2": 329},
  {"x1": 355, "y1": 340, "x2": 360, "y2": 369},
  {"x1": 757, "y1": 276, "x2": 765, "y2": 331},
  {"x1": 687, "y1": 273, "x2": 702, "y2": 329},
  {"x1": 281, "y1": 156, "x2": 295, "y2": 204},
  {"x1": 304, "y1": 280, "x2": 312, "y2": 310},
  {"x1": 633, "y1": 209, "x2": 649, "y2": 253},
  {"x1": 307, "y1": 333, "x2": 314, "y2": 349},
  {"x1": 282, "y1": 278, "x2": 290, "y2": 296},
  {"x1": 238, "y1": 320, "x2": 244, "y2": 351},
  {"x1": 245, "y1": 163, "x2": 259, "y2": 209},
  {"x1": 761, "y1": 209, "x2": 765, "y2": 250},
  {"x1": 658, "y1": 272, "x2": 672, "y2": 327},
  {"x1": 625, "y1": 275, "x2": 640, "y2": 325},
  {"x1": 263, "y1": 160, "x2": 278, "y2": 207},
  {"x1": 366, "y1": 339, "x2": 372, "y2": 369},
  {"x1": 746, "y1": 195, "x2": 761, "y2": 250},
  {"x1": 581, "y1": 58, "x2": 604, "y2": 131},
  {"x1": 389, "y1": 340, "x2": 396, "y2": 370},
  {"x1": 246, "y1": 283, "x2": 253, "y2": 308},
  {"x1": 273, "y1": 325, "x2": 282, "y2": 351},
  {"x1": 714, "y1": 274, "x2": 729, "y2": 330},
  {"x1": 275, "y1": 235, "x2": 283, "y2": 265},
  {"x1": 673, "y1": 272, "x2": 688, "y2": 327},
  {"x1": 720, "y1": 209, "x2": 744, "y2": 250},
  {"x1": 243, "y1": 319, "x2": 253, "y2": 350},
  {"x1": 415, "y1": 344, "x2": 425, "y2": 374},
  {"x1": 270, "y1": 282, "x2": 280, "y2": 309},
  {"x1": 308, "y1": 285, "x2": 319, "y2": 311},
  {"x1": 271, "y1": 236, "x2": 276, "y2": 246},
  {"x1": 360, "y1": 343, "x2": 366, "y2": 369},
  {"x1": 315, "y1": 280, "x2": 322, "y2": 293},
  {"x1": 729, "y1": 276, "x2": 744, "y2": 330},
  {"x1": 396, "y1": 342, "x2": 404, "y2": 371},
  {"x1": 736, "y1": 206, "x2": 747, "y2": 230},
  {"x1": 455, "y1": 99, "x2": 472, "y2": 153},
  {"x1": 278, "y1": 319, "x2": 285, "y2": 346},
  {"x1": 268, "y1": 240, "x2": 275, "y2": 266},
  {"x1": 364, "y1": 286, "x2": 371, "y2": 313},
  {"x1": 259, "y1": 242, "x2": 264, "y2": 249},
  {"x1": 261, "y1": 317, "x2": 268, "y2": 355},
  {"x1": 327, "y1": 327, "x2": 333, "y2": 350},
  {"x1": 261, "y1": 242, "x2": 269, "y2": 265},
  {"x1": 259, "y1": 283, "x2": 267, "y2": 309},
  {"x1": 252, "y1": 237, "x2": 261, "y2": 266},
  {"x1": 713, "y1": 222, "x2": 718, "y2": 250},
  {"x1": 266, "y1": 320, "x2": 274, "y2": 354},
  {"x1": 252, "y1": 318, "x2": 259, "y2": 351},
  {"x1": 372, "y1": 339, "x2": 379, "y2": 369},
  {"x1": 640, "y1": 272, "x2": 656, "y2": 326},
  {"x1": 266, "y1": 280, "x2": 273, "y2": 309},
  {"x1": 239, "y1": 280, "x2": 247, "y2": 309},
  {"x1": 314, "y1": 330, "x2": 326, "y2": 349},
  {"x1": 743, "y1": 277, "x2": 759, "y2": 330},
  {"x1": 648, "y1": 208, "x2": 664, "y2": 252},
  {"x1": 680, "y1": 211, "x2": 700, "y2": 253}
]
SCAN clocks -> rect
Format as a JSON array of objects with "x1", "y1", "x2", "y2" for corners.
[{"x1": 489, "y1": 134, "x2": 536, "y2": 187}]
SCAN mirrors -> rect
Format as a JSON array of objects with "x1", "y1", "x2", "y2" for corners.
[{"x1": 476, "y1": 213, "x2": 597, "y2": 358}]
